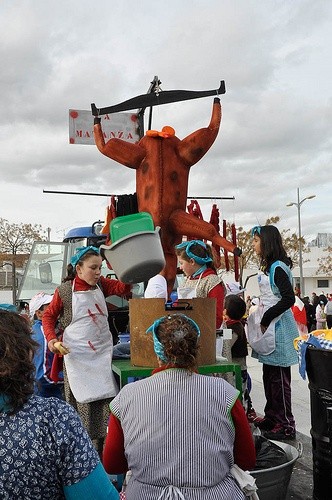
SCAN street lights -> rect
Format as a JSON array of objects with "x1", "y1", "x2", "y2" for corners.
[{"x1": 286, "y1": 186, "x2": 316, "y2": 298}]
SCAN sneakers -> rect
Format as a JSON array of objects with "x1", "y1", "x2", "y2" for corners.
[
  {"x1": 254, "y1": 417, "x2": 267, "y2": 427},
  {"x1": 264, "y1": 423, "x2": 296, "y2": 440}
]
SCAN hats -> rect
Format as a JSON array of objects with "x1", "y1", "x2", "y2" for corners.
[{"x1": 28, "y1": 292, "x2": 54, "y2": 320}]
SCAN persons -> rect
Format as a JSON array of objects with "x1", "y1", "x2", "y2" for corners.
[
  {"x1": 43, "y1": 246, "x2": 140, "y2": 457},
  {"x1": 240, "y1": 294, "x2": 259, "y2": 344},
  {"x1": 222, "y1": 295, "x2": 253, "y2": 427},
  {"x1": 292, "y1": 291, "x2": 332, "y2": 341},
  {"x1": 101, "y1": 313, "x2": 257, "y2": 500},
  {"x1": 249, "y1": 224, "x2": 304, "y2": 442},
  {"x1": 0, "y1": 308, "x2": 120, "y2": 500},
  {"x1": 172, "y1": 240, "x2": 227, "y2": 330},
  {"x1": 0, "y1": 291, "x2": 64, "y2": 400}
]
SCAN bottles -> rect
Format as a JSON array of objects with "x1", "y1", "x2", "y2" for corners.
[{"x1": 95, "y1": 224, "x2": 102, "y2": 235}]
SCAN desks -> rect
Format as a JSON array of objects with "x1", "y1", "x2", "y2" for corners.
[{"x1": 111, "y1": 356, "x2": 243, "y2": 407}]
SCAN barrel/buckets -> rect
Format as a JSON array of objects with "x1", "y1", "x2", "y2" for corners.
[
  {"x1": 249, "y1": 439, "x2": 303, "y2": 500},
  {"x1": 99, "y1": 227, "x2": 166, "y2": 285}
]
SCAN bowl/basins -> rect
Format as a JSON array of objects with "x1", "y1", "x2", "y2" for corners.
[
  {"x1": 62, "y1": 226, "x2": 98, "y2": 243},
  {"x1": 118, "y1": 334, "x2": 130, "y2": 343}
]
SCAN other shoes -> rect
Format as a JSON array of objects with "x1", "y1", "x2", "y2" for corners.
[{"x1": 247, "y1": 410, "x2": 258, "y2": 423}]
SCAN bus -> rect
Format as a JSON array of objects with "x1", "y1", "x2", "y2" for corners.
[{"x1": 14, "y1": 226, "x2": 187, "y2": 319}]
